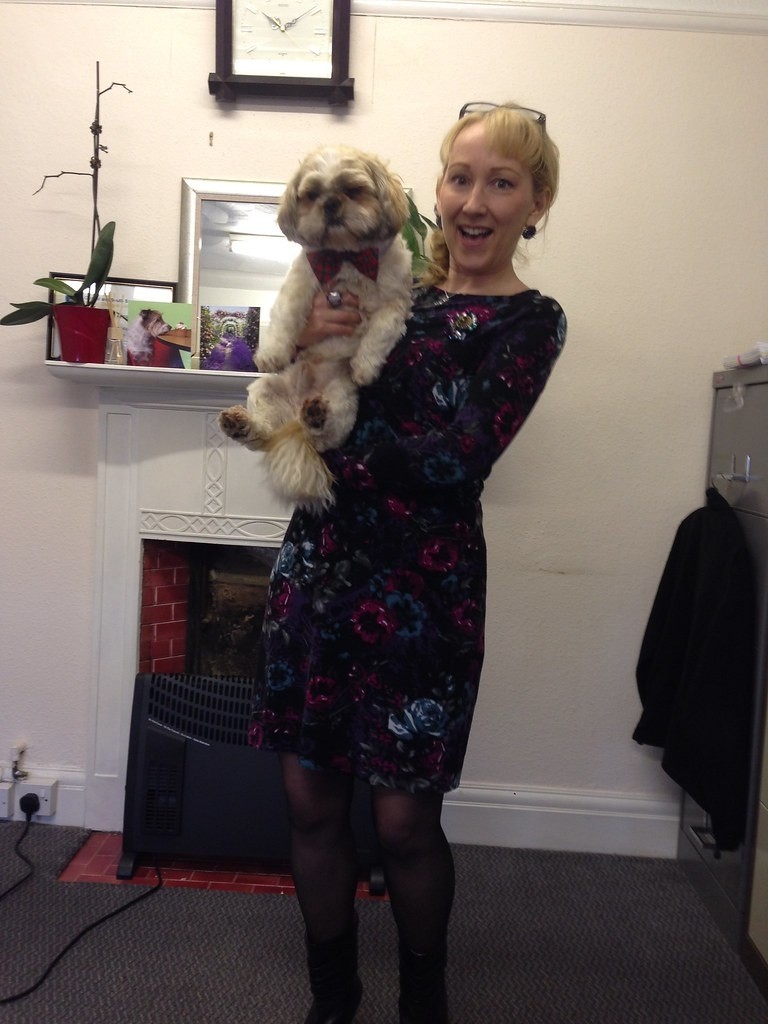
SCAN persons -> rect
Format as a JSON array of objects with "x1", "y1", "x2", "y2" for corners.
[{"x1": 254, "y1": 101, "x2": 568, "y2": 1024}]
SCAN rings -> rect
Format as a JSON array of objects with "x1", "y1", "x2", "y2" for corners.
[{"x1": 328, "y1": 291, "x2": 341, "y2": 307}]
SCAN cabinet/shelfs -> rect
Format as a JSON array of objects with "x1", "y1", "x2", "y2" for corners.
[{"x1": 675, "y1": 364, "x2": 767, "y2": 955}]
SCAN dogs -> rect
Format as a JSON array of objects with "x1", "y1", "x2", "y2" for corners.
[
  {"x1": 127, "y1": 308, "x2": 172, "y2": 366},
  {"x1": 217, "y1": 140, "x2": 415, "y2": 515}
]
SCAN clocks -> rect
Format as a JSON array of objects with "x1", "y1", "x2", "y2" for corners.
[{"x1": 209, "y1": 0, "x2": 355, "y2": 107}]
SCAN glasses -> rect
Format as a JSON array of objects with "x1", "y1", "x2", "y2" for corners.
[{"x1": 458, "y1": 101, "x2": 548, "y2": 146}]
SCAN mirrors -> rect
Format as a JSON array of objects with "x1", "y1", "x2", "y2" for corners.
[{"x1": 177, "y1": 178, "x2": 305, "y2": 368}]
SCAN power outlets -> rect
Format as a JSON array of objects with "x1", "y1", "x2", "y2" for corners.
[{"x1": 15, "y1": 779, "x2": 58, "y2": 816}]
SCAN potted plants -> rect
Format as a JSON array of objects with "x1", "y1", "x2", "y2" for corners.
[{"x1": 0, "y1": 220, "x2": 115, "y2": 364}]
